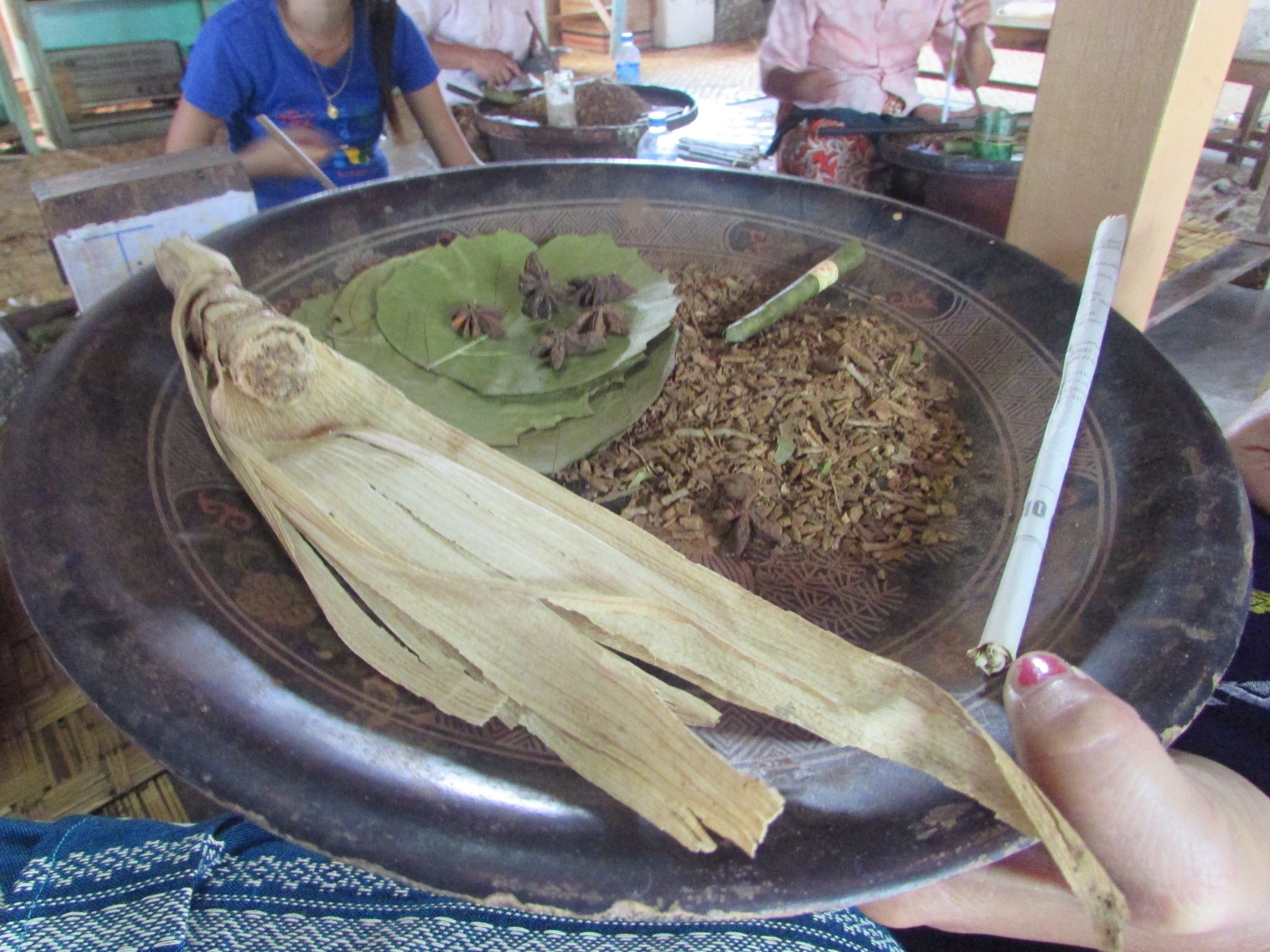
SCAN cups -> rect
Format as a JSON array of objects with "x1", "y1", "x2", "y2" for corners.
[
  {"x1": 545, "y1": 69, "x2": 576, "y2": 127},
  {"x1": 973, "y1": 110, "x2": 1020, "y2": 160}
]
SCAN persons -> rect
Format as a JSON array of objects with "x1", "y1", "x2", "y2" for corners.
[
  {"x1": 758, "y1": 0, "x2": 995, "y2": 191},
  {"x1": 849, "y1": 652, "x2": 1270, "y2": 952},
  {"x1": 166, "y1": 0, "x2": 485, "y2": 227},
  {"x1": 395, "y1": 0, "x2": 560, "y2": 141}
]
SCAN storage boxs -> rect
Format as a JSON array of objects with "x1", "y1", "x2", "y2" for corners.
[{"x1": 32, "y1": 144, "x2": 253, "y2": 313}]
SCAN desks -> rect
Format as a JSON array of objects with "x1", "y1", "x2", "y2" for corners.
[{"x1": 904, "y1": 0, "x2": 1270, "y2": 188}]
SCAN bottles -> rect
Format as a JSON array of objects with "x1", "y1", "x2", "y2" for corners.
[{"x1": 616, "y1": 32, "x2": 640, "y2": 81}]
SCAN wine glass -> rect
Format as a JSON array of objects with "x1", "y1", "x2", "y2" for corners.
[{"x1": 637, "y1": 110, "x2": 679, "y2": 160}]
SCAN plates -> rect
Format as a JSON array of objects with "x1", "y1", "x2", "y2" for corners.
[{"x1": 1, "y1": 158, "x2": 1252, "y2": 919}]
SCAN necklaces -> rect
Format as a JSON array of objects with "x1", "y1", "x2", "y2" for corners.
[{"x1": 294, "y1": 12, "x2": 355, "y2": 119}]
